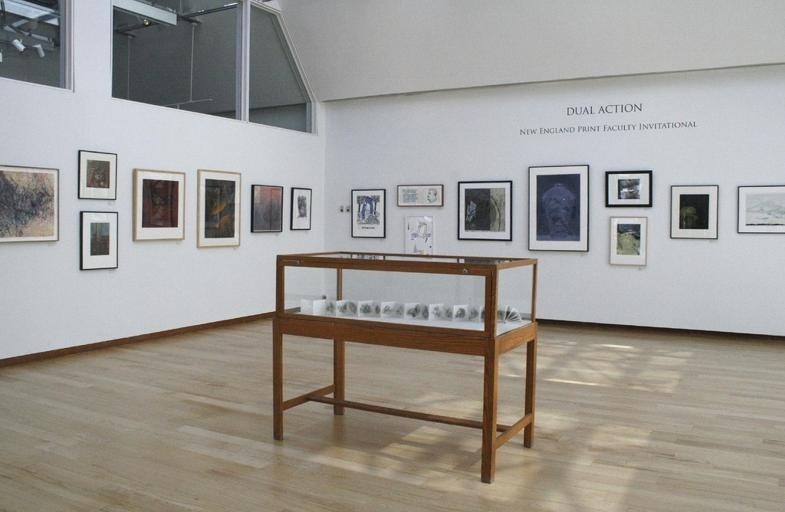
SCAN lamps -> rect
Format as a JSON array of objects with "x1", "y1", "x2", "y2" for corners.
[
  {"x1": 33, "y1": 43, "x2": 45, "y2": 57},
  {"x1": 12, "y1": 39, "x2": 26, "y2": 52}
]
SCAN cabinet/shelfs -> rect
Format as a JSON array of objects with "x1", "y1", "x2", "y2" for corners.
[{"x1": 272, "y1": 252, "x2": 539, "y2": 483}]
